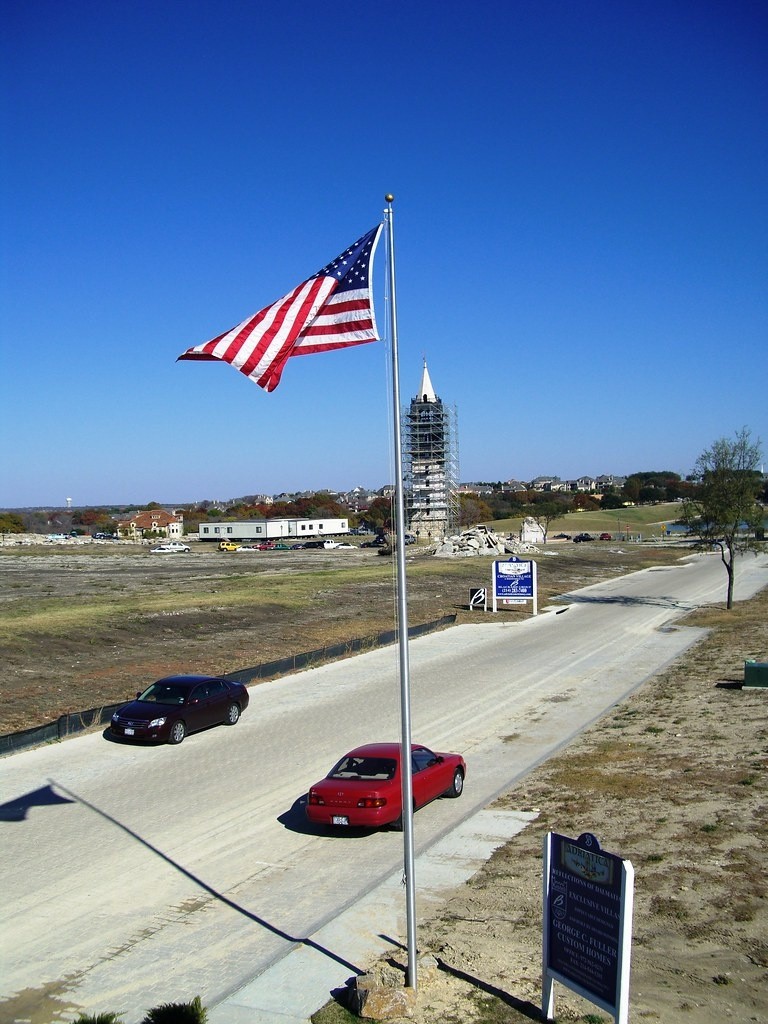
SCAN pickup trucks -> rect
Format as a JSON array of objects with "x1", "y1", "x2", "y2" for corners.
[{"x1": 160, "y1": 541, "x2": 191, "y2": 552}]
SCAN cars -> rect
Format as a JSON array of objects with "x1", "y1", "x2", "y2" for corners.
[
  {"x1": 93, "y1": 533, "x2": 115, "y2": 540},
  {"x1": 290, "y1": 543, "x2": 306, "y2": 550},
  {"x1": 305, "y1": 743, "x2": 466, "y2": 832},
  {"x1": 599, "y1": 532, "x2": 612, "y2": 541},
  {"x1": 240, "y1": 541, "x2": 291, "y2": 550},
  {"x1": 149, "y1": 547, "x2": 177, "y2": 553},
  {"x1": 553, "y1": 533, "x2": 571, "y2": 540},
  {"x1": 335, "y1": 542, "x2": 358, "y2": 550},
  {"x1": 108, "y1": 674, "x2": 249, "y2": 746},
  {"x1": 404, "y1": 534, "x2": 415, "y2": 546},
  {"x1": 573, "y1": 533, "x2": 593, "y2": 542}
]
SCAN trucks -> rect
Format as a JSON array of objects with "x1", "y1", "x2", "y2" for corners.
[{"x1": 323, "y1": 540, "x2": 344, "y2": 549}]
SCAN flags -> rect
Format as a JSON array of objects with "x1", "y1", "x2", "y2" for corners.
[{"x1": 172, "y1": 224, "x2": 383, "y2": 394}]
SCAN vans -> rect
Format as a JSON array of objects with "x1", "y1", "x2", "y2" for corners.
[{"x1": 304, "y1": 541, "x2": 325, "y2": 549}]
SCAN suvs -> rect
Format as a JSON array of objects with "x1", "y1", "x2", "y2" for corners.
[{"x1": 218, "y1": 541, "x2": 242, "y2": 551}]
